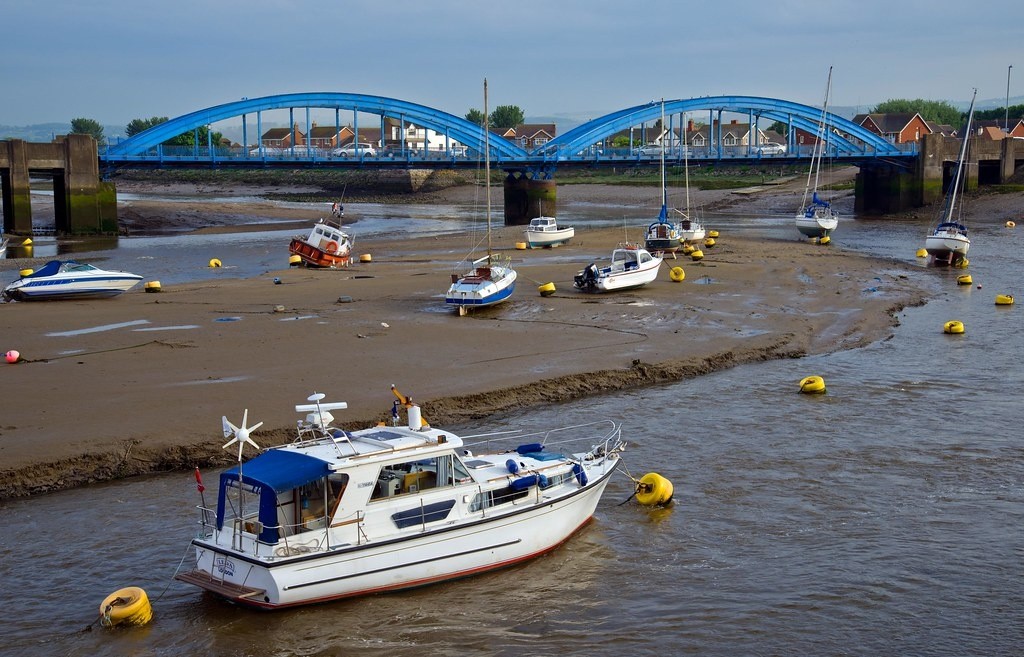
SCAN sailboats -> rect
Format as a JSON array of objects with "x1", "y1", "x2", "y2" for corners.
[
  {"x1": 443, "y1": 78, "x2": 519, "y2": 318},
  {"x1": 643, "y1": 97, "x2": 681, "y2": 253},
  {"x1": 794, "y1": 66, "x2": 843, "y2": 235},
  {"x1": 924, "y1": 88, "x2": 981, "y2": 264},
  {"x1": 673, "y1": 111, "x2": 706, "y2": 241}
]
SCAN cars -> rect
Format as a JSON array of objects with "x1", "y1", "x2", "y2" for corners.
[
  {"x1": 693, "y1": 146, "x2": 718, "y2": 156},
  {"x1": 382, "y1": 147, "x2": 418, "y2": 158},
  {"x1": 632, "y1": 144, "x2": 669, "y2": 155},
  {"x1": 751, "y1": 141, "x2": 787, "y2": 156}
]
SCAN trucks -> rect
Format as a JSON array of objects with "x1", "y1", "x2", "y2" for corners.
[{"x1": 451, "y1": 149, "x2": 468, "y2": 158}]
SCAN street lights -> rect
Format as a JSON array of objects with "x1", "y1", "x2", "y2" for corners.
[{"x1": 1005, "y1": 65, "x2": 1015, "y2": 133}]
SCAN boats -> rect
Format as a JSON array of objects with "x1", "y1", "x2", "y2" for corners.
[
  {"x1": 0, "y1": 259, "x2": 144, "y2": 303},
  {"x1": 522, "y1": 198, "x2": 575, "y2": 248},
  {"x1": 573, "y1": 213, "x2": 665, "y2": 295},
  {"x1": 287, "y1": 183, "x2": 355, "y2": 270},
  {"x1": 177, "y1": 385, "x2": 627, "y2": 611}
]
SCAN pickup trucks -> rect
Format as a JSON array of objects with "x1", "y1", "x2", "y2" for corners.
[{"x1": 331, "y1": 142, "x2": 376, "y2": 157}]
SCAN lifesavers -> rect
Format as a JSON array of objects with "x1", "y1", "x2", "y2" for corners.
[
  {"x1": 326, "y1": 241, "x2": 338, "y2": 253},
  {"x1": 625, "y1": 247, "x2": 637, "y2": 250}
]
